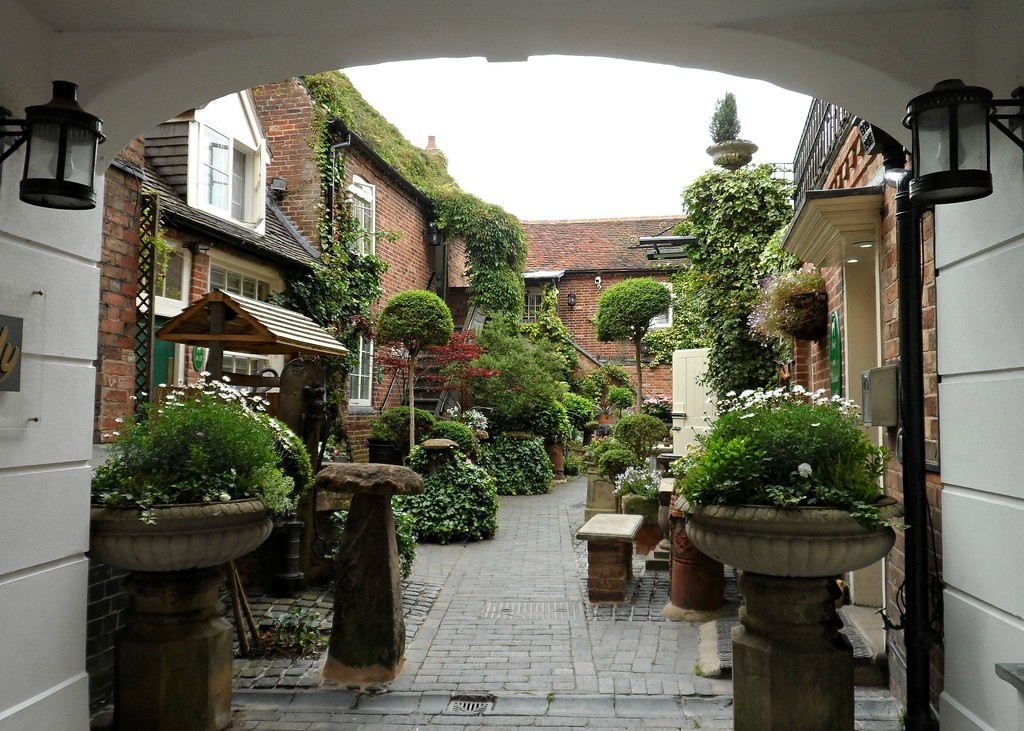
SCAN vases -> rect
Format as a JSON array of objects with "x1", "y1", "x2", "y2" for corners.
[
  {"x1": 674, "y1": 494, "x2": 904, "y2": 577},
  {"x1": 85, "y1": 498, "x2": 274, "y2": 572}
]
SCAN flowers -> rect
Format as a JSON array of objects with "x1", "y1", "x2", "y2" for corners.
[
  {"x1": 90, "y1": 371, "x2": 315, "y2": 526},
  {"x1": 663, "y1": 384, "x2": 909, "y2": 533}
]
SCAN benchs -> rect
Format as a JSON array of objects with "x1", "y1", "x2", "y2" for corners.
[{"x1": 576, "y1": 514, "x2": 645, "y2": 603}]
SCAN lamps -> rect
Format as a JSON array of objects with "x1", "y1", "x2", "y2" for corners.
[
  {"x1": 568, "y1": 294, "x2": 576, "y2": 306},
  {"x1": 0, "y1": 80, "x2": 106, "y2": 210},
  {"x1": 902, "y1": 79, "x2": 1024, "y2": 204},
  {"x1": 851, "y1": 116, "x2": 906, "y2": 189},
  {"x1": 271, "y1": 177, "x2": 286, "y2": 202}
]
